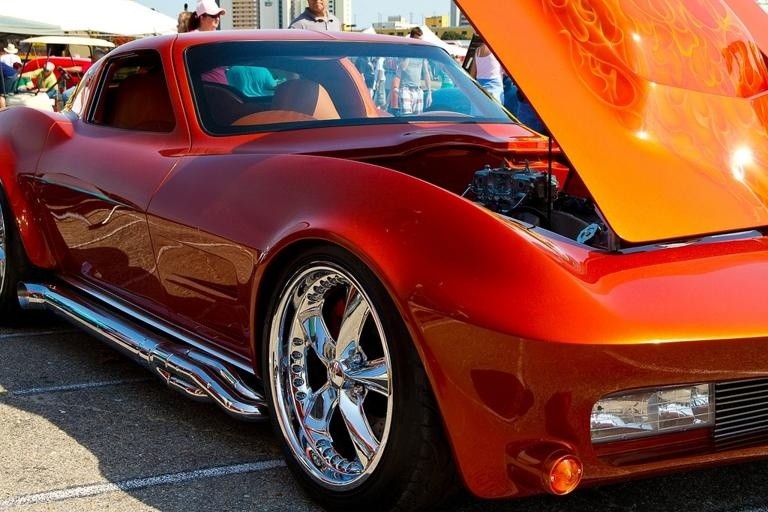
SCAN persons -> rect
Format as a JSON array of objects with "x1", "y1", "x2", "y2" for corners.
[
  {"x1": 187, "y1": 0, "x2": 228, "y2": 85},
  {"x1": 287, "y1": 0, "x2": 341, "y2": 32},
  {"x1": 352, "y1": 26, "x2": 432, "y2": 117},
  {"x1": 226, "y1": 65, "x2": 287, "y2": 99},
  {"x1": 469, "y1": 39, "x2": 506, "y2": 106},
  {"x1": 0, "y1": 42, "x2": 106, "y2": 118},
  {"x1": 176, "y1": 11, "x2": 192, "y2": 33}
]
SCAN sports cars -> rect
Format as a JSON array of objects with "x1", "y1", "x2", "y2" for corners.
[{"x1": 0, "y1": 1, "x2": 768, "y2": 506}]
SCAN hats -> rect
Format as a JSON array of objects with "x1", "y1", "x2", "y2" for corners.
[
  {"x1": 44, "y1": 61, "x2": 55, "y2": 73},
  {"x1": 194, "y1": 0, "x2": 226, "y2": 19},
  {"x1": 3, "y1": 43, "x2": 19, "y2": 54}
]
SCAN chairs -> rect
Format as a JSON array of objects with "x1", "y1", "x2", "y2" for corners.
[{"x1": 108, "y1": 74, "x2": 344, "y2": 131}]
